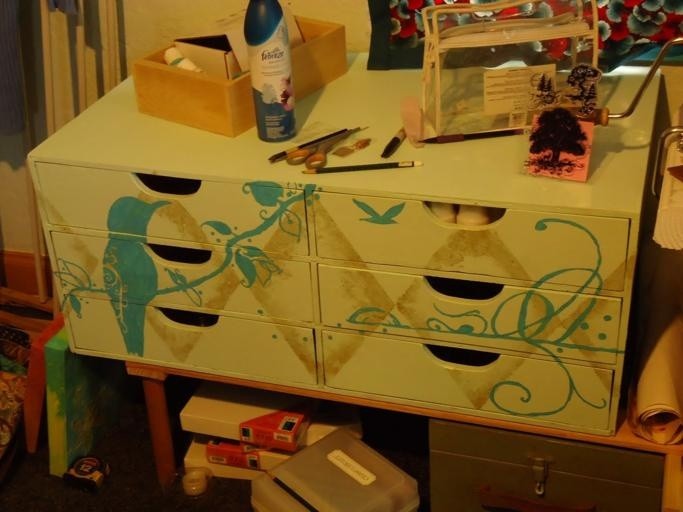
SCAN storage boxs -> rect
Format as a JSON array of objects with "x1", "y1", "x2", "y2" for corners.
[
  {"x1": 429, "y1": 420, "x2": 668, "y2": 512},
  {"x1": 132, "y1": 13, "x2": 347, "y2": 138}
]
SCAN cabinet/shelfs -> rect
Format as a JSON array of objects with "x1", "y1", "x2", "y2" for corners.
[{"x1": 25, "y1": 54, "x2": 661, "y2": 436}]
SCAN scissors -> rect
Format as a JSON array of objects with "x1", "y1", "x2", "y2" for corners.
[{"x1": 287, "y1": 128, "x2": 359, "y2": 167}]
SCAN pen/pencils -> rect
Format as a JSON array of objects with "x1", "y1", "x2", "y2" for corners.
[
  {"x1": 416, "y1": 129, "x2": 526, "y2": 143},
  {"x1": 302, "y1": 162, "x2": 423, "y2": 174},
  {"x1": 267, "y1": 128, "x2": 348, "y2": 163},
  {"x1": 381, "y1": 127, "x2": 406, "y2": 158}
]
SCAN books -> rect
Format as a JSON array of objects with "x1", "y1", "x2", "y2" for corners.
[{"x1": 179, "y1": 382, "x2": 375, "y2": 481}]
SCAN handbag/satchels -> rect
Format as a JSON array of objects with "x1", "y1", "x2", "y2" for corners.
[{"x1": 413, "y1": 0, "x2": 601, "y2": 138}]
